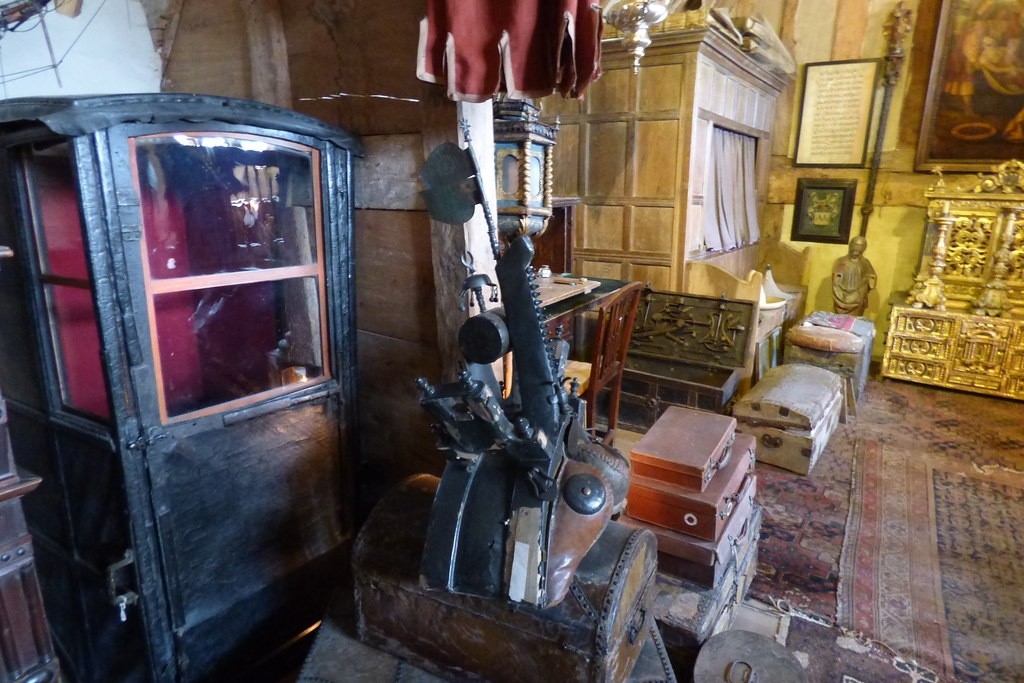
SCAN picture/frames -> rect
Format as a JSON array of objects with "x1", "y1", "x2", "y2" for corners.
[
  {"x1": 791, "y1": 177, "x2": 858, "y2": 243},
  {"x1": 913, "y1": 0, "x2": 1024, "y2": 173}
]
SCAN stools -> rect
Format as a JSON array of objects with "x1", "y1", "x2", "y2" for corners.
[{"x1": 784, "y1": 310, "x2": 877, "y2": 404}]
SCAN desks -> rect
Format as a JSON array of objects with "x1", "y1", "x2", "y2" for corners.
[{"x1": 534, "y1": 275, "x2": 634, "y2": 325}]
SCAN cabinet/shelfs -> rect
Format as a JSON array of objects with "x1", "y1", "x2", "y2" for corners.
[{"x1": 537, "y1": 23, "x2": 789, "y2": 299}]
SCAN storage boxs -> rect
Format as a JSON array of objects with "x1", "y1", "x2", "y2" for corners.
[{"x1": 297, "y1": 358, "x2": 844, "y2": 682}]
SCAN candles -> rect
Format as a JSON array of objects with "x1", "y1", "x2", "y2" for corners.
[
  {"x1": 1005, "y1": 217, "x2": 1016, "y2": 236},
  {"x1": 943, "y1": 200, "x2": 950, "y2": 213}
]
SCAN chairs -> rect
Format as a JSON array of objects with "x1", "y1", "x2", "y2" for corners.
[{"x1": 558, "y1": 280, "x2": 643, "y2": 447}]
